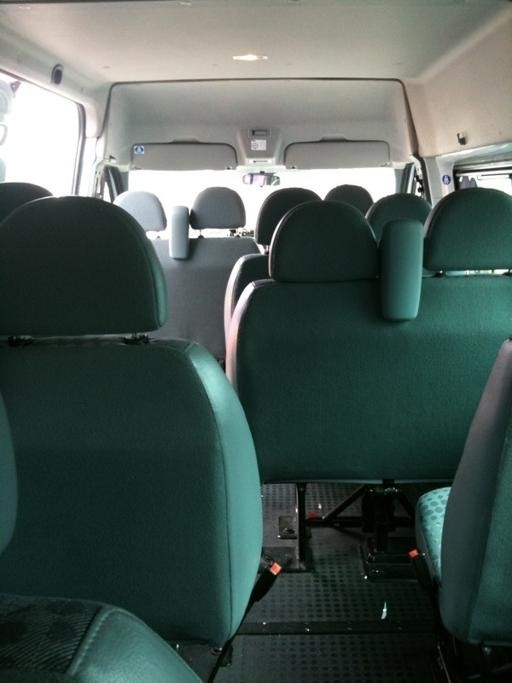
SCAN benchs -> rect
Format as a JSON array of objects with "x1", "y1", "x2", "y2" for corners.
[{"x1": 1, "y1": 180, "x2": 512, "y2": 683}]
